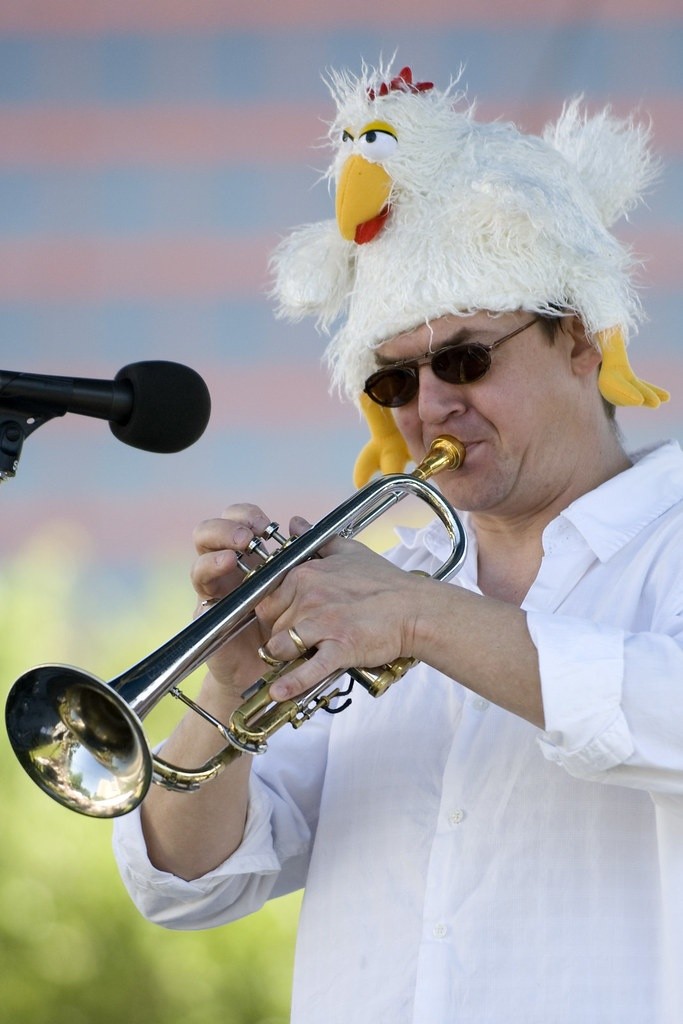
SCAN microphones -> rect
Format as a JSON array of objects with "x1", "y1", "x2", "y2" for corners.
[{"x1": 0, "y1": 359, "x2": 211, "y2": 454}]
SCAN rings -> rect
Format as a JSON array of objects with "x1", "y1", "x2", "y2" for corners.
[
  {"x1": 257, "y1": 639, "x2": 287, "y2": 667},
  {"x1": 288, "y1": 625, "x2": 308, "y2": 658}
]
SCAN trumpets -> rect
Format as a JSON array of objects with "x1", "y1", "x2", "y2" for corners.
[{"x1": 4, "y1": 433, "x2": 468, "y2": 820}]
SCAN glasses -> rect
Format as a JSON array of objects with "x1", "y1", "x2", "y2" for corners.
[{"x1": 363, "y1": 305, "x2": 560, "y2": 408}]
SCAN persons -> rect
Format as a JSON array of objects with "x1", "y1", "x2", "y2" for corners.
[{"x1": 111, "y1": 47, "x2": 682, "y2": 1024}]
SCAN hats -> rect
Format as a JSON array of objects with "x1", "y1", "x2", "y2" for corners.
[{"x1": 274, "y1": 65, "x2": 670, "y2": 494}]
